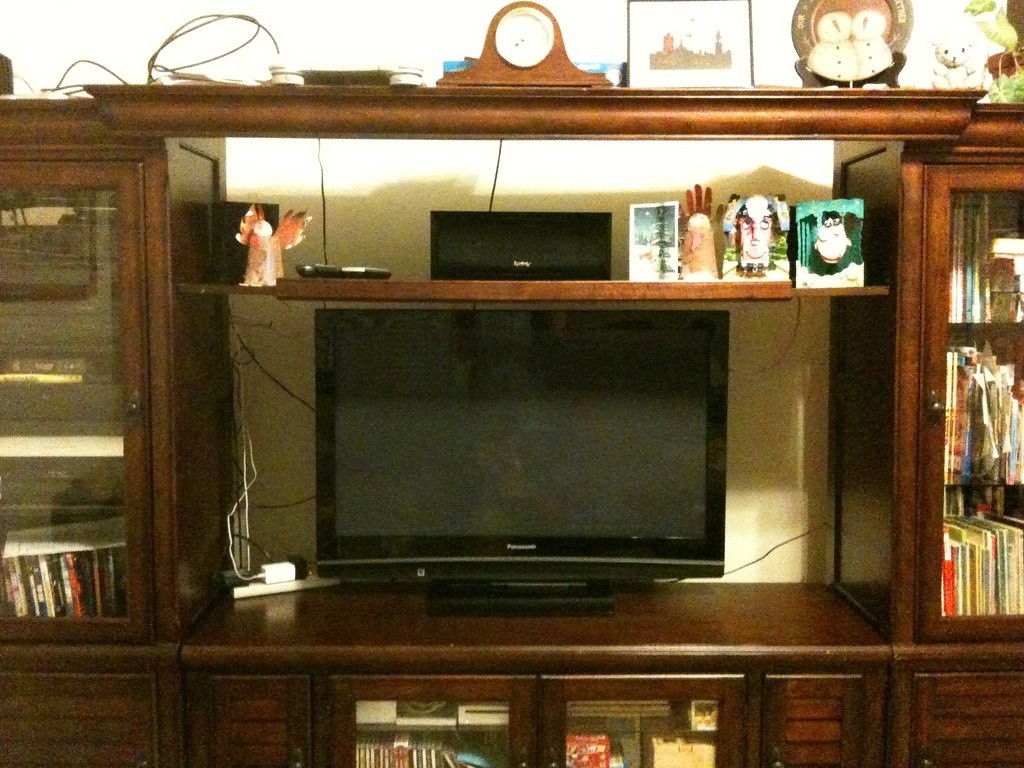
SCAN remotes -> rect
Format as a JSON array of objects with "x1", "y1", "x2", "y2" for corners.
[{"x1": 294, "y1": 263, "x2": 390, "y2": 280}]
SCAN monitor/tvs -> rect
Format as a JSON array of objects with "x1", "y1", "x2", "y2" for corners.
[{"x1": 314, "y1": 307, "x2": 729, "y2": 616}]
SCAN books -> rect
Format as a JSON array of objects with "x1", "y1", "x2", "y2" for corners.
[
  {"x1": 941, "y1": 486, "x2": 1024, "y2": 617},
  {"x1": 944, "y1": 338, "x2": 1024, "y2": 485},
  {"x1": 0, "y1": 358, "x2": 87, "y2": 384},
  {"x1": 356, "y1": 733, "x2": 492, "y2": 768},
  {"x1": 948, "y1": 189, "x2": 1024, "y2": 323},
  {"x1": 0, "y1": 514, "x2": 128, "y2": 618}
]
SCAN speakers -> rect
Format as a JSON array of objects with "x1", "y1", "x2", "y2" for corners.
[{"x1": 429, "y1": 210, "x2": 612, "y2": 281}]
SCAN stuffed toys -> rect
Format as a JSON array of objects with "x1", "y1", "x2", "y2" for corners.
[{"x1": 930, "y1": 40, "x2": 976, "y2": 90}]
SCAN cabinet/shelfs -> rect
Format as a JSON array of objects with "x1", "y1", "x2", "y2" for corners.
[{"x1": 0, "y1": 86, "x2": 1023, "y2": 767}]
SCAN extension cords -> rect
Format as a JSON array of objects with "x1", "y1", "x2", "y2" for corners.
[{"x1": 231, "y1": 572, "x2": 339, "y2": 601}]
